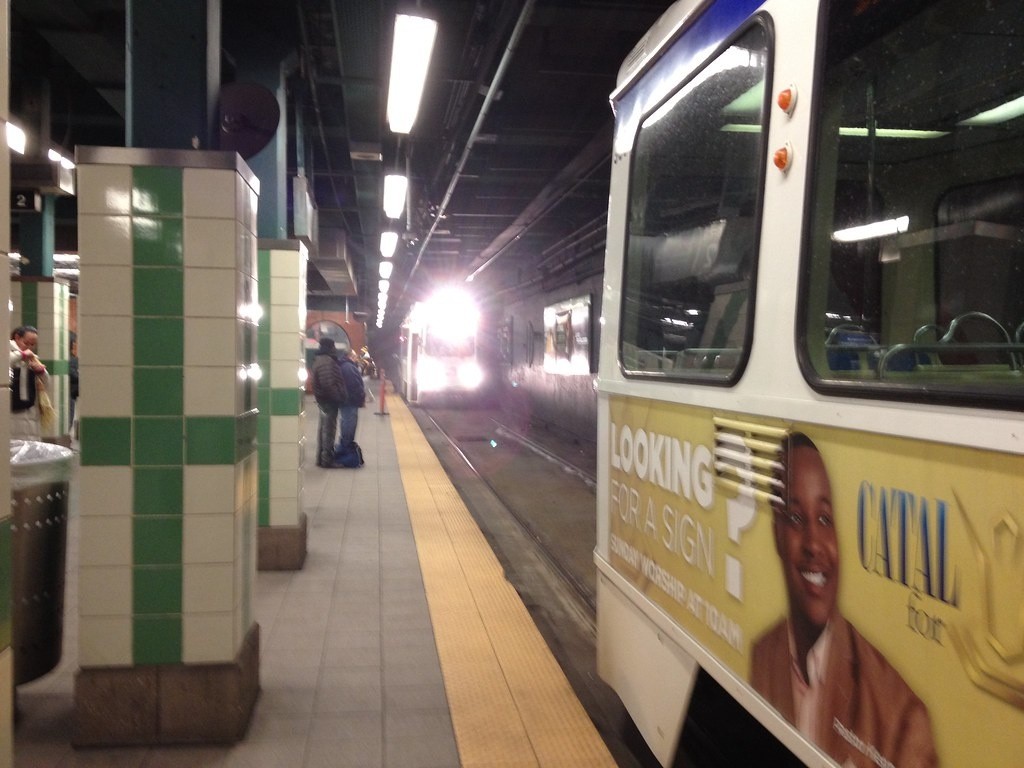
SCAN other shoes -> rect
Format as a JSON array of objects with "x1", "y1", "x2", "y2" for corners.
[{"x1": 326, "y1": 463, "x2": 345, "y2": 468}]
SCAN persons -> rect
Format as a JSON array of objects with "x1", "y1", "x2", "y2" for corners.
[
  {"x1": 69, "y1": 343, "x2": 79, "y2": 437},
  {"x1": 340, "y1": 349, "x2": 367, "y2": 440},
  {"x1": 748, "y1": 433, "x2": 938, "y2": 768},
  {"x1": 9, "y1": 326, "x2": 50, "y2": 438},
  {"x1": 356, "y1": 345, "x2": 376, "y2": 403},
  {"x1": 311, "y1": 337, "x2": 348, "y2": 468}
]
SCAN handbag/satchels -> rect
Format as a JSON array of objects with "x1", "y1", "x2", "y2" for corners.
[{"x1": 333, "y1": 444, "x2": 364, "y2": 468}]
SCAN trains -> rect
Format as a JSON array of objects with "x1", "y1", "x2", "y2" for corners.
[
  {"x1": 395, "y1": 285, "x2": 491, "y2": 408},
  {"x1": 565, "y1": 0, "x2": 1024, "y2": 768}
]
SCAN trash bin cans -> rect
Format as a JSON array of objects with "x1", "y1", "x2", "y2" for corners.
[{"x1": 10, "y1": 438, "x2": 78, "y2": 684}]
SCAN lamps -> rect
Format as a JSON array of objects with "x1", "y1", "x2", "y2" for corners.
[{"x1": 385, "y1": 0, "x2": 440, "y2": 135}]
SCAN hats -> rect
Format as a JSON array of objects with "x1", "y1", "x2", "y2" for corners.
[{"x1": 320, "y1": 338, "x2": 335, "y2": 351}]
